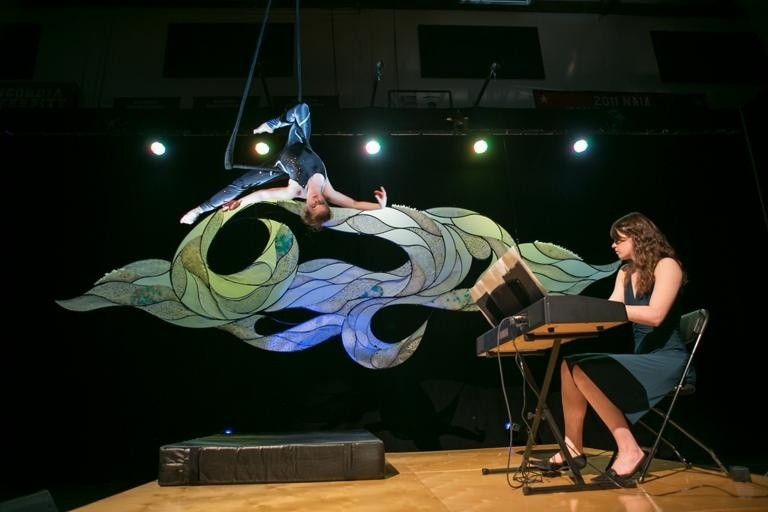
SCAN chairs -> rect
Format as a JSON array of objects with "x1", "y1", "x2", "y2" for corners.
[{"x1": 606, "y1": 309, "x2": 728, "y2": 483}]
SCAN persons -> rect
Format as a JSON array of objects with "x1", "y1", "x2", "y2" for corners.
[
  {"x1": 180, "y1": 102, "x2": 387, "y2": 227},
  {"x1": 536, "y1": 209, "x2": 697, "y2": 480}
]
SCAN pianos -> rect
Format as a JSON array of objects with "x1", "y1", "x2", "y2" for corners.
[{"x1": 477, "y1": 295, "x2": 627, "y2": 357}]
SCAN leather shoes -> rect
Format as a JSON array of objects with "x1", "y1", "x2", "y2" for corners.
[
  {"x1": 536, "y1": 453, "x2": 588, "y2": 472},
  {"x1": 590, "y1": 450, "x2": 650, "y2": 483}
]
